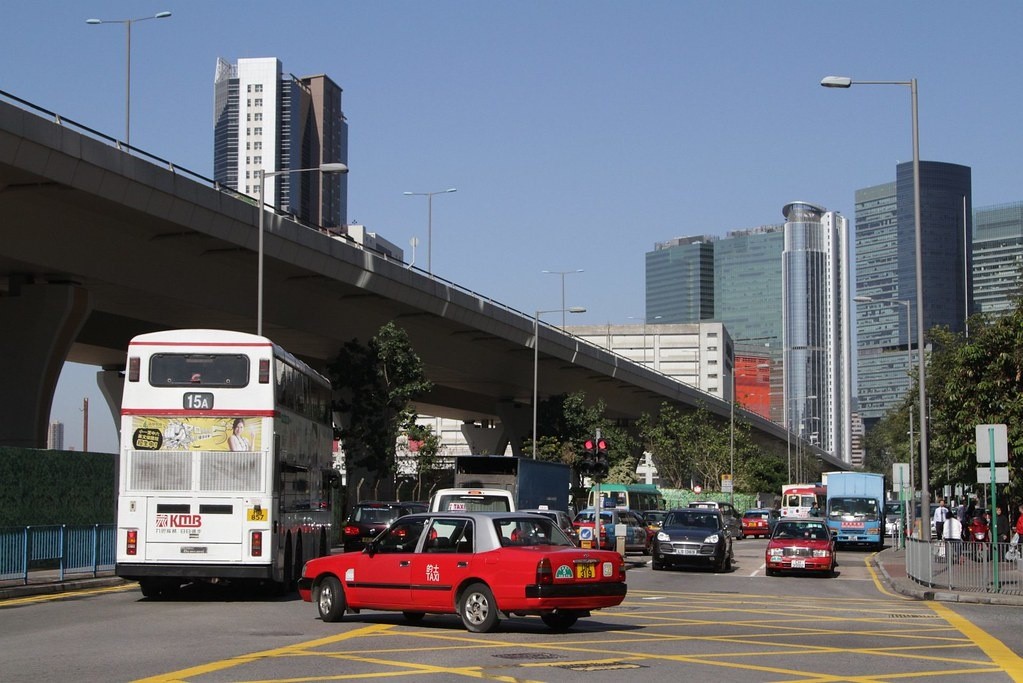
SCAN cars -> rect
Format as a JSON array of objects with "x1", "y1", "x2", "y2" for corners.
[
  {"x1": 297, "y1": 511, "x2": 629, "y2": 633},
  {"x1": 884, "y1": 498, "x2": 952, "y2": 541},
  {"x1": 572, "y1": 499, "x2": 781, "y2": 551},
  {"x1": 651, "y1": 507, "x2": 732, "y2": 572},
  {"x1": 766, "y1": 519, "x2": 837, "y2": 574}
]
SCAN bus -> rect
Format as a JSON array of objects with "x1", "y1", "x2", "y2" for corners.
[
  {"x1": 780, "y1": 487, "x2": 827, "y2": 518},
  {"x1": 113, "y1": 327, "x2": 342, "y2": 600},
  {"x1": 586, "y1": 485, "x2": 666, "y2": 512}
]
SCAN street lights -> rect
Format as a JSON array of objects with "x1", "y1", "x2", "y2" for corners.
[
  {"x1": 542, "y1": 270, "x2": 584, "y2": 333},
  {"x1": 730, "y1": 364, "x2": 770, "y2": 505},
  {"x1": 628, "y1": 315, "x2": 661, "y2": 369},
  {"x1": 786, "y1": 395, "x2": 820, "y2": 484},
  {"x1": 85, "y1": 11, "x2": 172, "y2": 152},
  {"x1": 852, "y1": 296, "x2": 916, "y2": 538},
  {"x1": 821, "y1": 76, "x2": 928, "y2": 544},
  {"x1": 533, "y1": 307, "x2": 586, "y2": 460},
  {"x1": 257, "y1": 163, "x2": 350, "y2": 337},
  {"x1": 404, "y1": 188, "x2": 457, "y2": 277}
]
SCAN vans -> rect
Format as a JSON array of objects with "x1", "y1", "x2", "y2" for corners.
[{"x1": 427, "y1": 487, "x2": 518, "y2": 549}]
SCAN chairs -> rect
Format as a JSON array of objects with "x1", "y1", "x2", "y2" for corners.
[
  {"x1": 675, "y1": 516, "x2": 688, "y2": 525},
  {"x1": 427, "y1": 537, "x2": 455, "y2": 553},
  {"x1": 704, "y1": 517, "x2": 716, "y2": 528},
  {"x1": 457, "y1": 530, "x2": 472, "y2": 552}
]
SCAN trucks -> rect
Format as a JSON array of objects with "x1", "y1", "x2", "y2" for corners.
[
  {"x1": 454, "y1": 454, "x2": 579, "y2": 527},
  {"x1": 826, "y1": 472, "x2": 886, "y2": 551}
]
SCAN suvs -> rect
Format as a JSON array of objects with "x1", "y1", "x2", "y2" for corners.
[{"x1": 338, "y1": 499, "x2": 430, "y2": 554}]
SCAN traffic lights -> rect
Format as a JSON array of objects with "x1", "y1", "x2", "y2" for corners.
[{"x1": 580, "y1": 437, "x2": 609, "y2": 478}]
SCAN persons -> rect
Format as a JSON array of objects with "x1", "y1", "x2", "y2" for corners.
[
  {"x1": 933, "y1": 499, "x2": 949, "y2": 541},
  {"x1": 228, "y1": 418, "x2": 255, "y2": 451},
  {"x1": 810, "y1": 502, "x2": 821, "y2": 518},
  {"x1": 1016, "y1": 504, "x2": 1023, "y2": 558},
  {"x1": 865, "y1": 504, "x2": 877, "y2": 516},
  {"x1": 779, "y1": 526, "x2": 791, "y2": 536},
  {"x1": 942, "y1": 512, "x2": 962, "y2": 565},
  {"x1": 989, "y1": 504, "x2": 1009, "y2": 562},
  {"x1": 957, "y1": 499, "x2": 976, "y2": 542}
]
